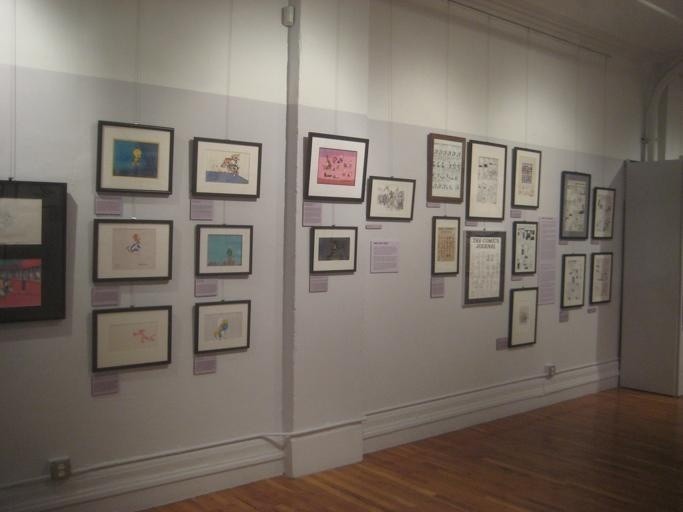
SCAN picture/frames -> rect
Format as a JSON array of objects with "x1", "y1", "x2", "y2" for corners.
[
  {"x1": 191, "y1": 136, "x2": 262, "y2": 198},
  {"x1": 194, "y1": 300, "x2": 252, "y2": 354},
  {"x1": 91, "y1": 305, "x2": 173, "y2": 371},
  {"x1": 366, "y1": 176, "x2": 417, "y2": 221},
  {"x1": 304, "y1": 132, "x2": 369, "y2": 202},
  {"x1": 95, "y1": 119, "x2": 175, "y2": 196},
  {"x1": 195, "y1": 224, "x2": 253, "y2": 276},
  {"x1": 559, "y1": 170, "x2": 617, "y2": 309},
  {"x1": 92, "y1": 218, "x2": 174, "y2": 282},
  {"x1": 0, "y1": 180, "x2": 67, "y2": 324},
  {"x1": 310, "y1": 225, "x2": 358, "y2": 273},
  {"x1": 426, "y1": 132, "x2": 541, "y2": 349}
]
